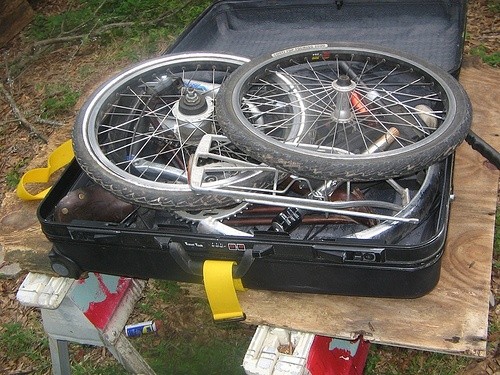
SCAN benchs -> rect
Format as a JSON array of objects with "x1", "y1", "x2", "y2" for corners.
[{"x1": 40, "y1": 267, "x2": 158, "y2": 374}]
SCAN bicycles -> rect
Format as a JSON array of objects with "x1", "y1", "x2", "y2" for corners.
[{"x1": 73, "y1": 43, "x2": 472, "y2": 224}]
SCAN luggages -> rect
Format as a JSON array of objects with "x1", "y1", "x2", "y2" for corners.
[{"x1": 36, "y1": 0, "x2": 467, "y2": 324}]
